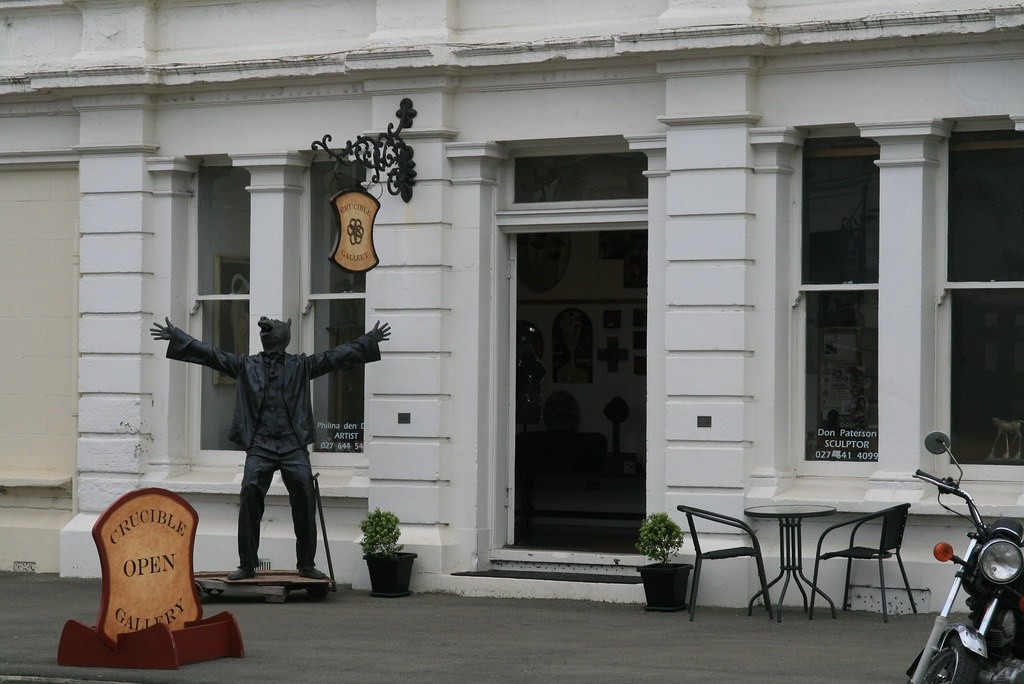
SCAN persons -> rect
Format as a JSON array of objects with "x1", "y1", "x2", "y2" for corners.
[{"x1": 148, "y1": 317, "x2": 390, "y2": 580}]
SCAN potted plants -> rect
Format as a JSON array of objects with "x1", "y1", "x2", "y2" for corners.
[
  {"x1": 604, "y1": 398, "x2": 638, "y2": 479},
  {"x1": 359, "y1": 507, "x2": 418, "y2": 597},
  {"x1": 636, "y1": 512, "x2": 694, "y2": 612}
]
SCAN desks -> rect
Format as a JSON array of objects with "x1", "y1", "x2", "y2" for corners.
[
  {"x1": 517, "y1": 431, "x2": 607, "y2": 477},
  {"x1": 744, "y1": 507, "x2": 836, "y2": 622}
]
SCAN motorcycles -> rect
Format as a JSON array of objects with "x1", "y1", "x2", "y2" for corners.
[{"x1": 905, "y1": 432, "x2": 1024, "y2": 684}]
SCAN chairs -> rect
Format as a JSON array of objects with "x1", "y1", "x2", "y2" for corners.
[
  {"x1": 677, "y1": 505, "x2": 774, "y2": 621},
  {"x1": 809, "y1": 503, "x2": 918, "y2": 622}
]
